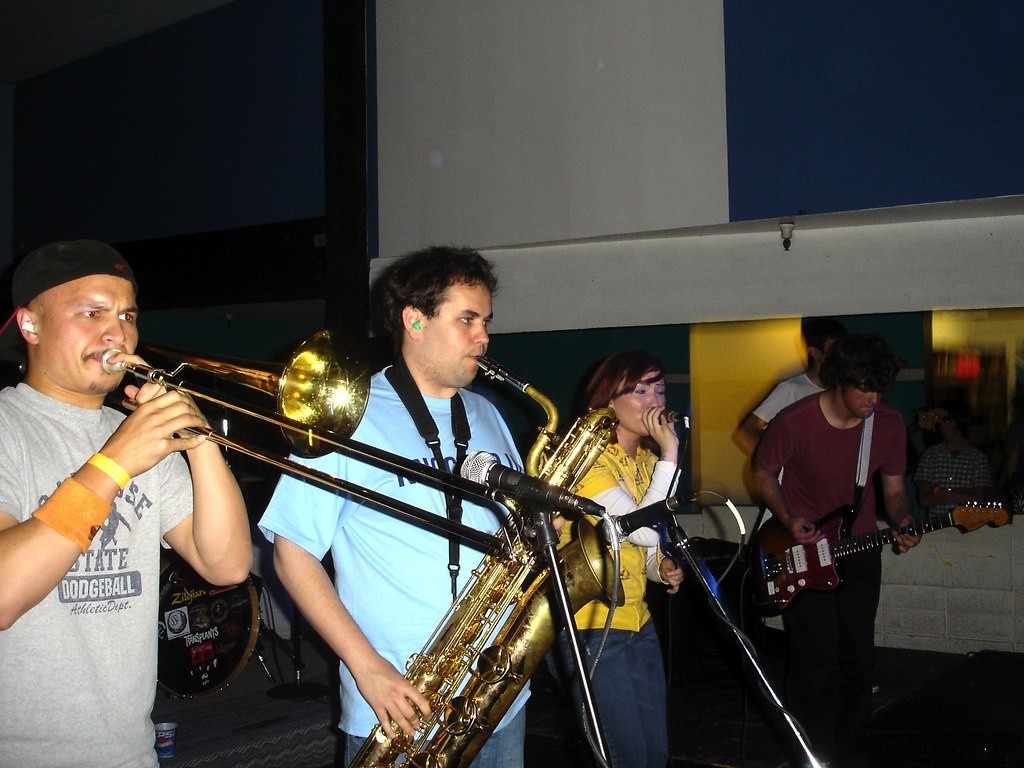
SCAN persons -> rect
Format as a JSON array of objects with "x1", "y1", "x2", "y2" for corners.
[
  {"x1": 258, "y1": 247, "x2": 530, "y2": 767},
  {"x1": 914, "y1": 414, "x2": 991, "y2": 508},
  {"x1": 556, "y1": 349, "x2": 685, "y2": 767},
  {"x1": 0, "y1": 241, "x2": 255, "y2": 768},
  {"x1": 732, "y1": 320, "x2": 848, "y2": 457},
  {"x1": 754, "y1": 333, "x2": 922, "y2": 768}
]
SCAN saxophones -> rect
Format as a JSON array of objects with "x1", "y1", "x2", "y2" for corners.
[{"x1": 347, "y1": 351, "x2": 624, "y2": 768}]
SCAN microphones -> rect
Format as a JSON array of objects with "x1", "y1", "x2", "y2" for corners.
[
  {"x1": 459, "y1": 450, "x2": 605, "y2": 517},
  {"x1": 599, "y1": 489, "x2": 697, "y2": 542},
  {"x1": 648, "y1": 407, "x2": 685, "y2": 425}
]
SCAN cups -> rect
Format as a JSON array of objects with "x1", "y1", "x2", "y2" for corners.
[{"x1": 154, "y1": 723, "x2": 177, "y2": 758}]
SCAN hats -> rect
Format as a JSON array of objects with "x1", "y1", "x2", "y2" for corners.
[{"x1": 0, "y1": 239, "x2": 138, "y2": 362}]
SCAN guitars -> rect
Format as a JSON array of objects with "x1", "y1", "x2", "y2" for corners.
[{"x1": 751, "y1": 498, "x2": 1010, "y2": 617}]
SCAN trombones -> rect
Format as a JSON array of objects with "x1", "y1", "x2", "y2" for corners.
[{"x1": 102, "y1": 326, "x2": 517, "y2": 561}]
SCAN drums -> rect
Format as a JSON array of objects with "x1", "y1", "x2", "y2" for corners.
[{"x1": 157, "y1": 552, "x2": 261, "y2": 702}]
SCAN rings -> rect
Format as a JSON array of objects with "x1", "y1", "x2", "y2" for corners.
[{"x1": 409, "y1": 715, "x2": 417, "y2": 721}]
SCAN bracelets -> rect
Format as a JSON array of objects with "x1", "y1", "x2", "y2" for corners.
[
  {"x1": 33, "y1": 478, "x2": 112, "y2": 554},
  {"x1": 87, "y1": 452, "x2": 129, "y2": 489}
]
